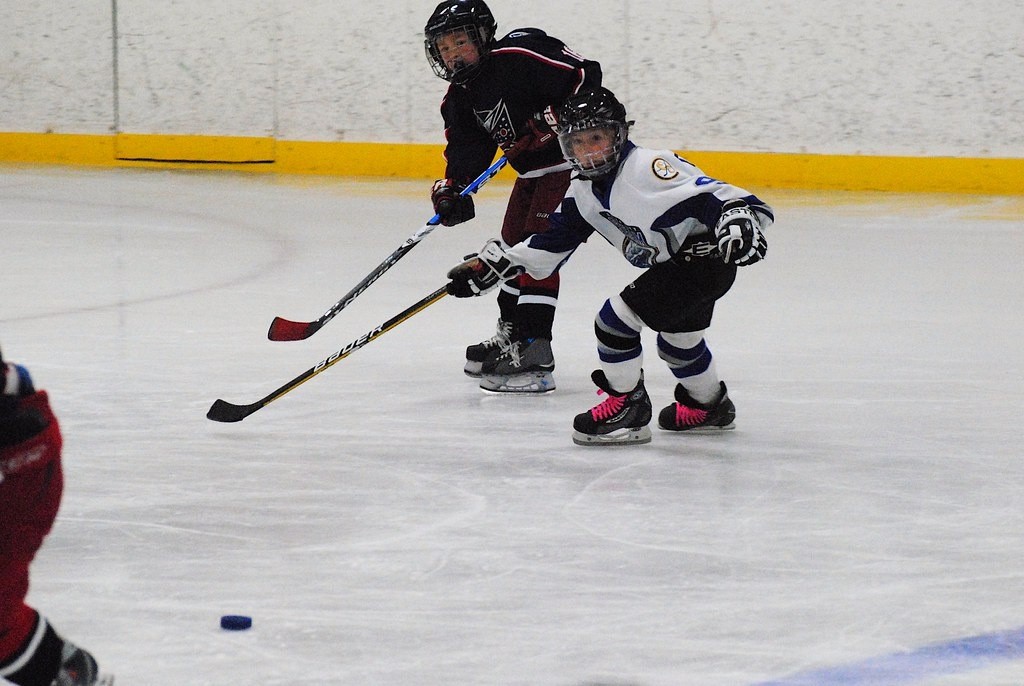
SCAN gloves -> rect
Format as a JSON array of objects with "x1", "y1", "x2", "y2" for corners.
[
  {"x1": 446, "y1": 239, "x2": 522, "y2": 298},
  {"x1": 715, "y1": 198, "x2": 768, "y2": 267},
  {"x1": 431, "y1": 177, "x2": 477, "y2": 228}
]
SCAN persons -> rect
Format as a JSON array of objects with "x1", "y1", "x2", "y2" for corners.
[
  {"x1": 0, "y1": 351, "x2": 114, "y2": 686},
  {"x1": 423, "y1": 0, "x2": 603, "y2": 393},
  {"x1": 447, "y1": 86, "x2": 774, "y2": 446}
]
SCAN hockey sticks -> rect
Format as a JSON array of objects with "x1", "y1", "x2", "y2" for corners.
[
  {"x1": 267, "y1": 151, "x2": 510, "y2": 342},
  {"x1": 205, "y1": 282, "x2": 454, "y2": 422}
]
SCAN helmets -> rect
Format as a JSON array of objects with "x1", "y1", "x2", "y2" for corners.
[
  {"x1": 425, "y1": 1, "x2": 497, "y2": 84},
  {"x1": 559, "y1": 88, "x2": 628, "y2": 176}
]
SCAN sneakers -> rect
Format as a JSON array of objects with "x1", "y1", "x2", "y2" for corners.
[
  {"x1": 659, "y1": 381, "x2": 736, "y2": 431},
  {"x1": 7, "y1": 614, "x2": 110, "y2": 686},
  {"x1": 572, "y1": 369, "x2": 654, "y2": 446},
  {"x1": 464, "y1": 317, "x2": 558, "y2": 394}
]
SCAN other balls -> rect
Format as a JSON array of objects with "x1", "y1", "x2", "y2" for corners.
[{"x1": 220, "y1": 615, "x2": 252, "y2": 631}]
